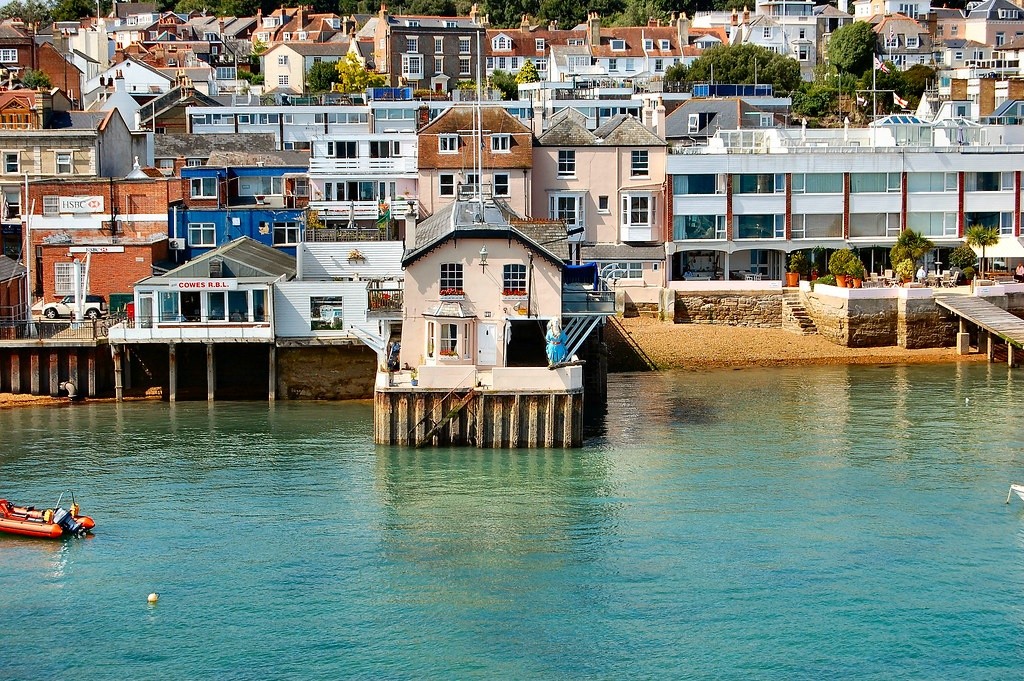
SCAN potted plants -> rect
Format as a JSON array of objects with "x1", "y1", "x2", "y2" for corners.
[
  {"x1": 897, "y1": 258, "x2": 918, "y2": 284},
  {"x1": 960, "y1": 267, "x2": 975, "y2": 285},
  {"x1": 784, "y1": 251, "x2": 819, "y2": 286},
  {"x1": 411, "y1": 373, "x2": 419, "y2": 385},
  {"x1": 828, "y1": 249, "x2": 864, "y2": 288}
]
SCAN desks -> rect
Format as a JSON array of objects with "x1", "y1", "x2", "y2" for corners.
[
  {"x1": 878, "y1": 277, "x2": 886, "y2": 286},
  {"x1": 732, "y1": 272, "x2": 737, "y2": 276},
  {"x1": 933, "y1": 275, "x2": 943, "y2": 288}
]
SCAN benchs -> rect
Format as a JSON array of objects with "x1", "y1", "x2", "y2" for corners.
[
  {"x1": 994, "y1": 275, "x2": 1019, "y2": 285},
  {"x1": 682, "y1": 271, "x2": 712, "y2": 281}
]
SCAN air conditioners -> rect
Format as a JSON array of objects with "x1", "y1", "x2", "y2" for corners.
[{"x1": 169, "y1": 238, "x2": 186, "y2": 249}]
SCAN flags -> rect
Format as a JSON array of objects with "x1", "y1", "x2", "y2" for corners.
[
  {"x1": 856, "y1": 94, "x2": 868, "y2": 107},
  {"x1": 873, "y1": 58, "x2": 891, "y2": 74},
  {"x1": 893, "y1": 93, "x2": 908, "y2": 109}
]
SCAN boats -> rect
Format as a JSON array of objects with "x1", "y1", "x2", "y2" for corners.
[{"x1": 0, "y1": 491, "x2": 96, "y2": 540}]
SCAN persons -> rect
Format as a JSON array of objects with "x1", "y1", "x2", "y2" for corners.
[
  {"x1": 176, "y1": 314, "x2": 187, "y2": 321},
  {"x1": 231, "y1": 309, "x2": 242, "y2": 319},
  {"x1": 1016, "y1": 263, "x2": 1024, "y2": 283},
  {"x1": 545, "y1": 317, "x2": 567, "y2": 367},
  {"x1": 917, "y1": 266, "x2": 926, "y2": 283},
  {"x1": 257, "y1": 304, "x2": 264, "y2": 321}
]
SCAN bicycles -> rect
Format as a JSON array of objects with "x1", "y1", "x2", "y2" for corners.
[{"x1": 101, "y1": 317, "x2": 112, "y2": 337}]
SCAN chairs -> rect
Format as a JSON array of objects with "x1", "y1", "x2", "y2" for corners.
[
  {"x1": 745, "y1": 273, "x2": 762, "y2": 281},
  {"x1": 739, "y1": 271, "x2": 755, "y2": 277},
  {"x1": 927, "y1": 270, "x2": 959, "y2": 288},
  {"x1": 864, "y1": 269, "x2": 900, "y2": 288}
]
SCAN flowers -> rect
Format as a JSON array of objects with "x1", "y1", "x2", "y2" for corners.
[
  {"x1": 503, "y1": 289, "x2": 527, "y2": 296},
  {"x1": 440, "y1": 288, "x2": 465, "y2": 295},
  {"x1": 440, "y1": 348, "x2": 459, "y2": 356},
  {"x1": 348, "y1": 249, "x2": 362, "y2": 259}
]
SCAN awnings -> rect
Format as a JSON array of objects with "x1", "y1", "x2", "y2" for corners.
[{"x1": 962, "y1": 236, "x2": 1024, "y2": 258}]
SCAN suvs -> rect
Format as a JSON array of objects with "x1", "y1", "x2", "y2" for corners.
[{"x1": 41, "y1": 293, "x2": 108, "y2": 320}]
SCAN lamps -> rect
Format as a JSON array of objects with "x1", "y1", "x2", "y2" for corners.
[{"x1": 479, "y1": 244, "x2": 488, "y2": 274}]
